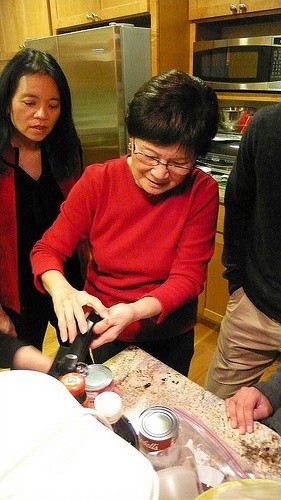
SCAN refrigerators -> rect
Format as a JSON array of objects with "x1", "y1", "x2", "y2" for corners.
[{"x1": 23, "y1": 22, "x2": 153, "y2": 174}]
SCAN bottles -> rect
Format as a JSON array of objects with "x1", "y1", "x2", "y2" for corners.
[
  {"x1": 92, "y1": 392, "x2": 138, "y2": 449},
  {"x1": 61, "y1": 372, "x2": 89, "y2": 408}
]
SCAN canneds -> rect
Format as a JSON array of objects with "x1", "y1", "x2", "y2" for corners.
[
  {"x1": 82, "y1": 364, "x2": 113, "y2": 408},
  {"x1": 138, "y1": 406, "x2": 180, "y2": 453}
]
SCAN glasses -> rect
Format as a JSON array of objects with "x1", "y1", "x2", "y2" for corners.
[{"x1": 130, "y1": 136, "x2": 197, "y2": 176}]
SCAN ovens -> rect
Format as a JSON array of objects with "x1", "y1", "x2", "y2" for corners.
[{"x1": 192, "y1": 35, "x2": 281, "y2": 93}]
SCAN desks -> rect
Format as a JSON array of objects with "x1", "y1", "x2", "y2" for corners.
[{"x1": 100, "y1": 345, "x2": 281, "y2": 483}]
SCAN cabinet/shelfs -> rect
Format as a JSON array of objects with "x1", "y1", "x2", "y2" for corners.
[
  {"x1": 49, "y1": 0, "x2": 151, "y2": 36},
  {"x1": 187, "y1": 0, "x2": 281, "y2": 24},
  {"x1": 197, "y1": 203, "x2": 229, "y2": 328}
]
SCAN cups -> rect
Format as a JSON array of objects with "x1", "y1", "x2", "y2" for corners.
[{"x1": 155, "y1": 462, "x2": 200, "y2": 500}]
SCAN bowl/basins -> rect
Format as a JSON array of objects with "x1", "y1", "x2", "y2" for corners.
[{"x1": 216, "y1": 105, "x2": 258, "y2": 135}]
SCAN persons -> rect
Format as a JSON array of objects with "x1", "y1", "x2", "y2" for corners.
[
  {"x1": 30, "y1": 70, "x2": 218, "y2": 378},
  {"x1": 1, "y1": 51, "x2": 85, "y2": 378},
  {"x1": 223, "y1": 366, "x2": 281, "y2": 435},
  {"x1": 204, "y1": 105, "x2": 281, "y2": 400}
]
